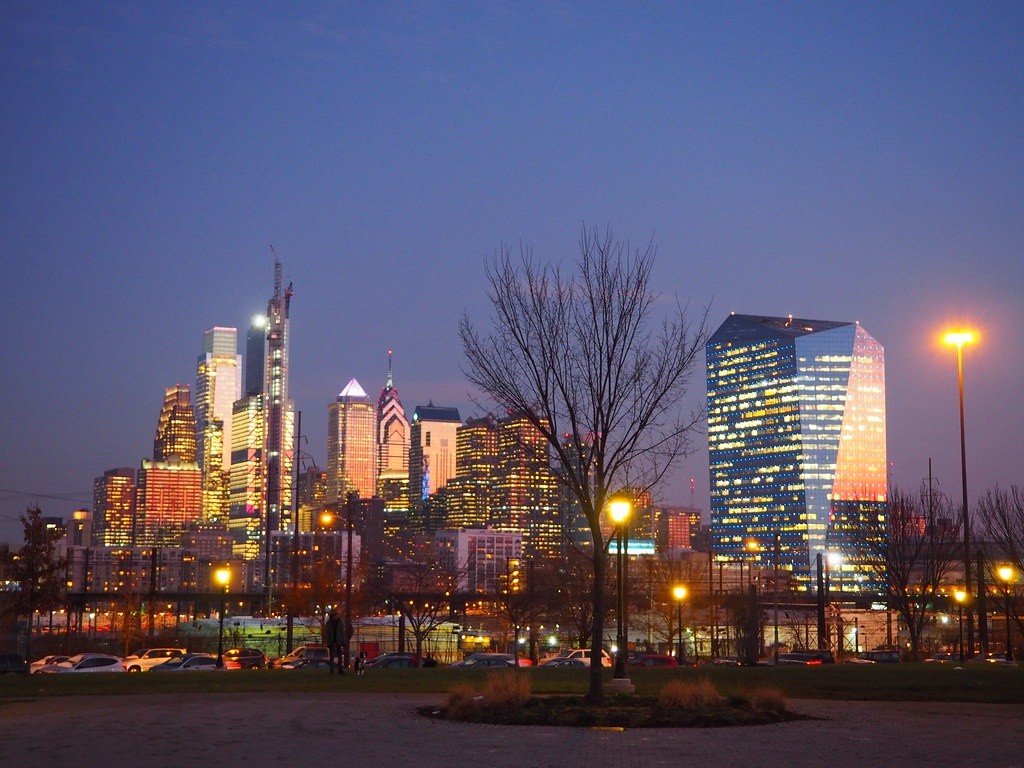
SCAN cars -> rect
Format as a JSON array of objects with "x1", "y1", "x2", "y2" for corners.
[
  {"x1": 974, "y1": 651, "x2": 1012, "y2": 663},
  {"x1": 33, "y1": 652, "x2": 127, "y2": 674},
  {"x1": 924, "y1": 652, "x2": 973, "y2": 662},
  {"x1": 625, "y1": 642, "x2": 905, "y2": 667},
  {"x1": 0, "y1": 654, "x2": 59, "y2": 674},
  {"x1": 148, "y1": 653, "x2": 228, "y2": 671},
  {"x1": 222, "y1": 643, "x2": 342, "y2": 672},
  {"x1": 364, "y1": 653, "x2": 437, "y2": 668},
  {"x1": 446, "y1": 646, "x2": 613, "y2": 669}
]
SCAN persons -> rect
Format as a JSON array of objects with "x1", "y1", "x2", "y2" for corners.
[
  {"x1": 326, "y1": 611, "x2": 345, "y2": 673},
  {"x1": 354, "y1": 652, "x2": 368, "y2": 675}
]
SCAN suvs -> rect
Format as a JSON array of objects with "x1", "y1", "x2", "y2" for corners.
[{"x1": 122, "y1": 648, "x2": 187, "y2": 672}]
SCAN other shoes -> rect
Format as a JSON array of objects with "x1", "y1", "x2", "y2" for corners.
[
  {"x1": 339, "y1": 669, "x2": 345, "y2": 675},
  {"x1": 330, "y1": 670, "x2": 334, "y2": 673}
]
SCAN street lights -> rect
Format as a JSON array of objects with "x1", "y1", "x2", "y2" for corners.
[
  {"x1": 943, "y1": 329, "x2": 979, "y2": 663},
  {"x1": 609, "y1": 501, "x2": 632, "y2": 678},
  {"x1": 673, "y1": 586, "x2": 686, "y2": 668},
  {"x1": 322, "y1": 512, "x2": 352, "y2": 670},
  {"x1": 954, "y1": 589, "x2": 966, "y2": 665},
  {"x1": 999, "y1": 560, "x2": 1014, "y2": 662},
  {"x1": 215, "y1": 568, "x2": 230, "y2": 671}
]
